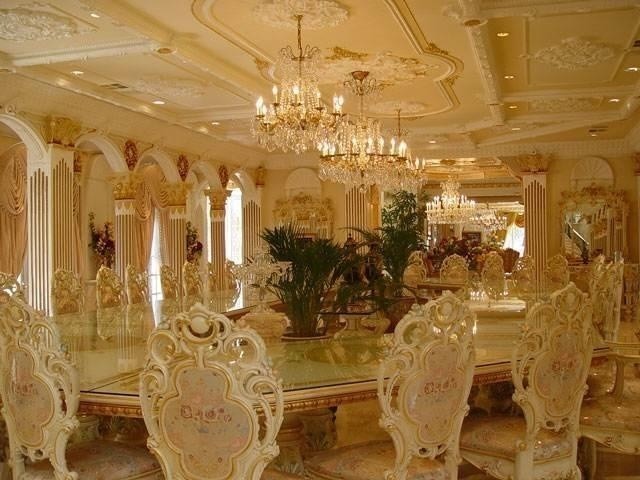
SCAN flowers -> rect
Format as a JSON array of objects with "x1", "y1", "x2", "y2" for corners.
[
  {"x1": 187, "y1": 223, "x2": 203, "y2": 260},
  {"x1": 88, "y1": 223, "x2": 116, "y2": 265}
]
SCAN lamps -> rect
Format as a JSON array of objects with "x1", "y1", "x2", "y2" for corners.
[
  {"x1": 395, "y1": 108, "x2": 507, "y2": 230},
  {"x1": 318, "y1": 72, "x2": 411, "y2": 190},
  {"x1": 250, "y1": 16, "x2": 346, "y2": 157}
]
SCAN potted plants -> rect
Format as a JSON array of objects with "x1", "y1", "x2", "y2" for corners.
[
  {"x1": 259, "y1": 224, "x2": 359, "y2": 341},
  {"x1": 358, "y1": 193, "x2": 428, "y2": 329}
]
